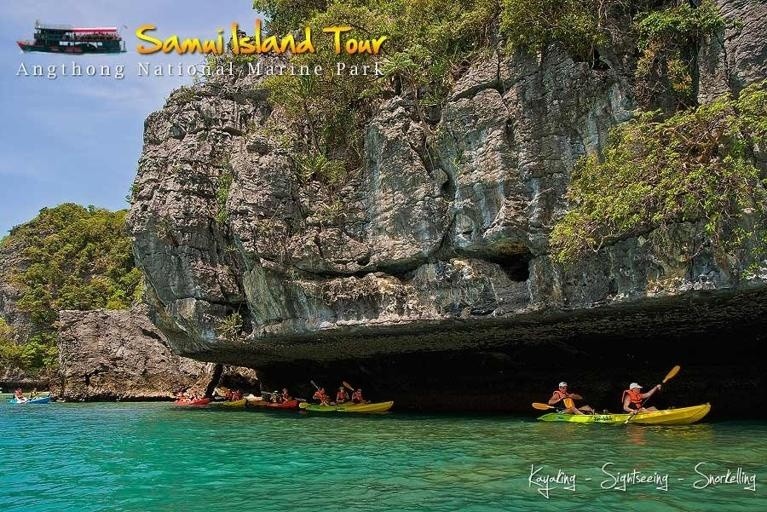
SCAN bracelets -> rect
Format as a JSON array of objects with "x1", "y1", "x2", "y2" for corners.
[{"x1": 557, "y1": 398, "x2": 561, "y2": 401}]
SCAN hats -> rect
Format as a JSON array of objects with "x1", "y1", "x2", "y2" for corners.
[
  {"x1": 629, "y1": 382, "x2": 644, "y2": 390},
  {"x1": 558, "y1": 382, "x2": 568, "y2": 390}
]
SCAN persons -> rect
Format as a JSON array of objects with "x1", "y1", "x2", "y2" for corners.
[
  {"x1": 350, "y1": 387, "x2": 371, "y2": 406},
  {"x1": 173, "y1": 377, "x2": 290, "y2": 408},
  {"x1": 29, "y1": 386, "x2": 41, "y2": 400},
  {"x1": 548, "y1": 381, "x2": 594, "y2": 416},
  {"x1": 14, "y1": 387, "x2": 26, "y2": 402},
  {"x1": 620, "y1": 382, "x2": 662, "y2": 414},
  {"x1": 334, "y1": 385, "x2": 349, "y2": 405},
  {"x1": 312, "y1": 387, "x2": 338, "y2": 407}
]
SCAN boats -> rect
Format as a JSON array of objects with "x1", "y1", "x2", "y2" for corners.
[
  {"x1": 173, "y1": 393, "x2": 393, "y2": 416},
  {"x1": 537, "y1": 401, "x2": 713, "y2": 426},
  {"x1": 9, "y1": 396, "x2": 51, "y2": 404}
]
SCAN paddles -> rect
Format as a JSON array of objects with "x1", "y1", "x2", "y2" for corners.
[
  {"x1": 343, "y1": 382, "x2": 355, "y2": 391},
  {"x1": 261, "y1": 390, "x2": 308, "y2": 402},
  {"x1": 532, "y1": 402, "x2": 567, "y2": 410},
  {"x1": 311, "y1": 380, "x2": 328, "y2": 405},
  {"x1": 623, "y1": 365, "x2": 680, "y2": 424}
]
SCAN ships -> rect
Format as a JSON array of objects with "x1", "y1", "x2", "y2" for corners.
[{"x1": 15, "y1": 18, "x2": 130, "y2": 56}]
